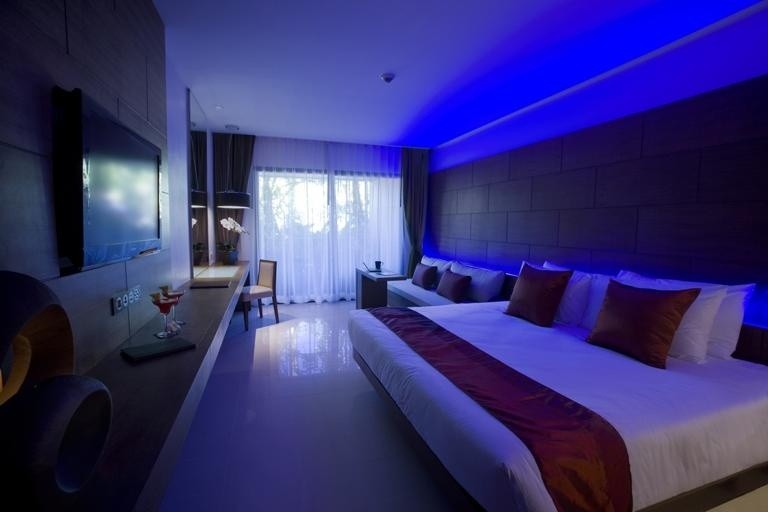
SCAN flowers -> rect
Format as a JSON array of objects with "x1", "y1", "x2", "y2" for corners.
[{"x1": 220, "y1": 217, "x2": 249, "y2": 251}]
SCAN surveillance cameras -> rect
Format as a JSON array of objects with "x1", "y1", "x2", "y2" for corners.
[{"x1": 381, "y1": 74, "x2": 395, "y2": 83}]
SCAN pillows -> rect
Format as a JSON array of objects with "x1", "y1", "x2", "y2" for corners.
[
  {"x1": 412, "y1": 255, "x2": 503, "y2": 304},
  {"x1": 503, "y1": 261, "x2": 755, "y2": 369}
]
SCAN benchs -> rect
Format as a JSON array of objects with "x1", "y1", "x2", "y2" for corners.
[{"x1": 387, "y1": 255, "x2": 519, "y2": 308}]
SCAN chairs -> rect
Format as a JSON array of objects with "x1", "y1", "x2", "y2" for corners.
[{"x1": 230, "y1": 259, "x2": 279, "y2": 331}]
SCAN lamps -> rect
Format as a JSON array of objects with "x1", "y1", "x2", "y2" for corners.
[
  {"x1": 215, "y1": 124, "x2": 251, "y2": 209},
  {"x1": 191, "y1": 122, "x2": 207, "y2": 209}
]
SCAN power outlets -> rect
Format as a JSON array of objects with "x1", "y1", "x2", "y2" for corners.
[{"x1": 110, "y1": 284, "x2": 144, "y2": 315}]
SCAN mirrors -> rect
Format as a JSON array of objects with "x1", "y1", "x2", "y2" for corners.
[{"x1": 187, "y1": 88, "x2": 209, "y2": 279}]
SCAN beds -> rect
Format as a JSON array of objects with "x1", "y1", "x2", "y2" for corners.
[{"x1": 349, "y1": 301, "x2": 768, "y2": 512}]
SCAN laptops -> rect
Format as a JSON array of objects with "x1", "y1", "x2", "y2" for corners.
[{"x1": 363, "y1": 262, "x2": 381, "y2": 272}]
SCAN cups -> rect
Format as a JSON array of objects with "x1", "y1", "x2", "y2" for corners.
[{"x1": 375, "y1": 261, "x2": 384, "y2": 269}]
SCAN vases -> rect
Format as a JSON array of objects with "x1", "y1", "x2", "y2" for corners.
[
  {"x1": 194, "y1": 252, "x2": 203, "y2": 266},
  {"x1": 224, "y1": 251, "x2": 238, "y2": 265}
]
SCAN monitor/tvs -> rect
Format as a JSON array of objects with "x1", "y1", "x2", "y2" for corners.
[{"x1": 61, "y1": 89, "x2": 162, "y2": 272}]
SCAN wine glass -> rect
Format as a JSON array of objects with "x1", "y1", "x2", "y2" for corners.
[
  {"x1": 162, "y1": 290, "x2": 185, "y2": 326},
  {"x1": 152, "y1": 298, "x2": 177, "y2": 338}
]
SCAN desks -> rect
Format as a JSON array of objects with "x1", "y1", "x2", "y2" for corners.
[{"x1": 356, "y1": 267, "x2": 407, "y2": 309}]
